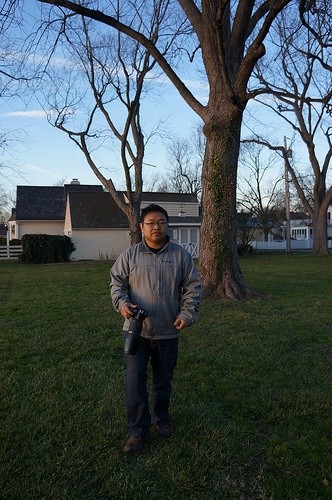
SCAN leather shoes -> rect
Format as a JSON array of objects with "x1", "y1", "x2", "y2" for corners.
[
  {"x1": 154, "y1": 422, "x2": 171, "y2": 438},
  {"x1": 123, "y1": 431, "x2": 151, "y2": 453}
]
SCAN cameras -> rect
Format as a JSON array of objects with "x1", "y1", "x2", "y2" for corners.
[{"x1": 124, "y1": 307, "x2": 149, "y2": 355}]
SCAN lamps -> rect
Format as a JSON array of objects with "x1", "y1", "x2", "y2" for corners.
[{"x1": 177, "y1": 201, "x2": 186, "y2": 218}]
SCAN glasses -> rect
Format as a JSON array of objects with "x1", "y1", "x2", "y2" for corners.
[{"x1": 142, "y1": 221, "x2": 169, "y2": 227}]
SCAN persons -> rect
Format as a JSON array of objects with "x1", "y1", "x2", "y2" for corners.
[{"x1": 108, "y1": 203, "x2": 201, "y2": 453}]
